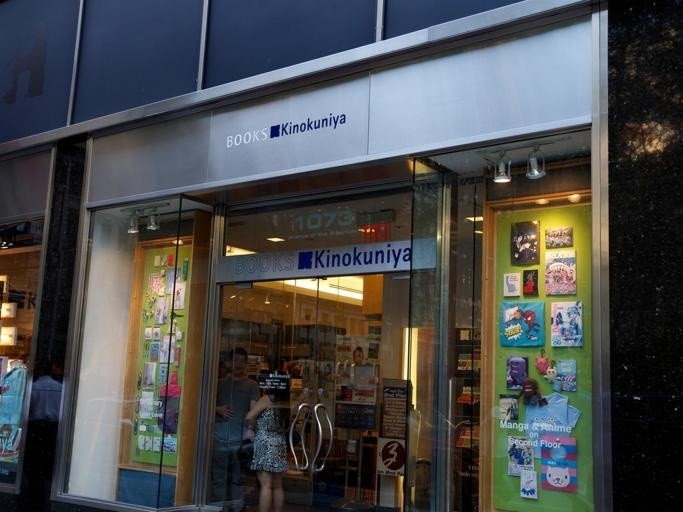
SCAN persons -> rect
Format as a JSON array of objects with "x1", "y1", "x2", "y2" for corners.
[
  {"x1": 245, "y1": 369, "x2": 291, "y2": 512},
  {"x1": 524, "y1": 271, "x2": 536, "y2": 294},
  {"x1": 513, "y1": 233, "x2": 526, "y2": 263},
  {"x1": 353, "y1": 346, "x2": 364, "y2": 364},
  {"x1": 509, "y1": 438, "x2": 532, "y2": 465},
  {"x1": 323, "y1": 365, "x2": 332, "y2": 381},
  {"x1": 209, "y1": 346, "x2": 260, "y2": 505},
  {"x1": 19, "y1": 361, "x2": 63, "y2": 512}
]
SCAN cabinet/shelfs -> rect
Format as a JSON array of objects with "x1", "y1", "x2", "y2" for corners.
[{"x1": 217, "y1": 316, "x2": 346, "y2": 381}]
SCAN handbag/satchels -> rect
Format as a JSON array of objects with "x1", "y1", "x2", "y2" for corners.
[
  {"x1": 285, "y1": 428, "x2": 299, "y2": 452},
  {"x1": 236, "y1": 439, "x2": 256, "y2": 477}
]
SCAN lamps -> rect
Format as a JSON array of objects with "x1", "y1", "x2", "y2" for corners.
[
  {"x1": 120, "y1": 200, "x2": 171, "y2": 236},
  {"x1": 475, "y1": 136, "x2": 556, "y2": 184}
]
